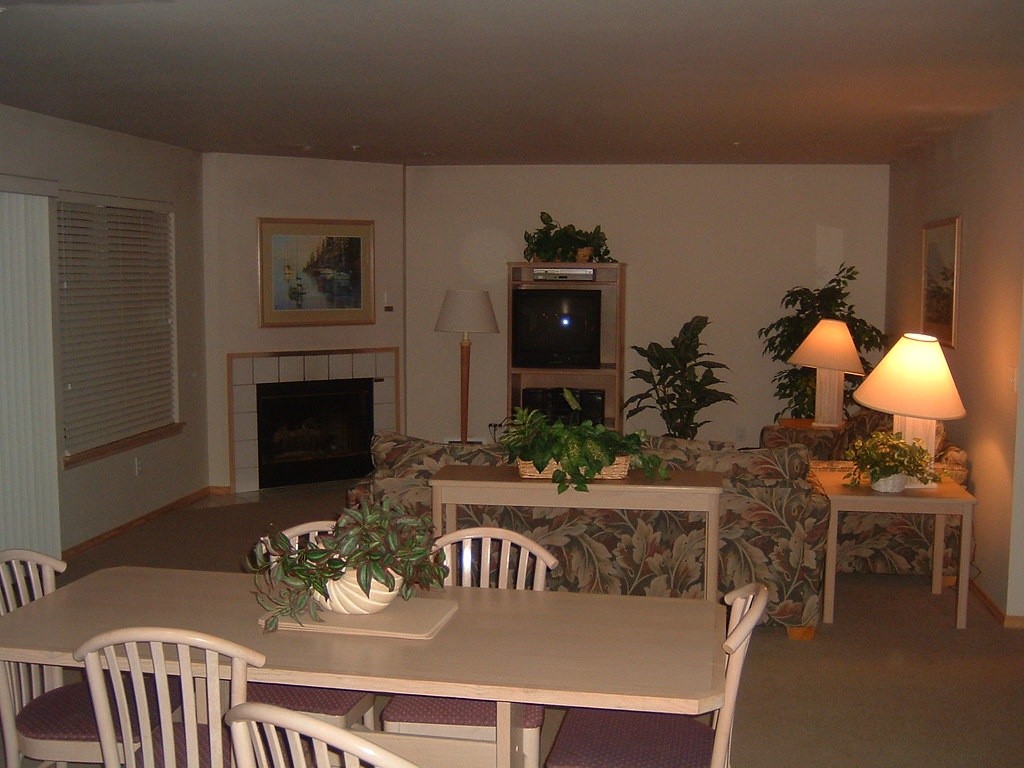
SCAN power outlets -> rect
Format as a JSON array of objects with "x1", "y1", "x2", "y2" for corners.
[{"x1": 488, "y1": 422, "x2": 503, "y2": 429}]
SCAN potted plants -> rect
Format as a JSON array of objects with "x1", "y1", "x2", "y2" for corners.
[
  {"x1": 499, "y1": 387, "x2": 672, "y2": 496},
  {"x1": 524, "y1": 210, "x2": 620, "y2": 264},
  {"x1": 843, "y1": 431, "x2": 944, "y2": 493},
  {"x1": 244, "y1": 491, "x2": 450, "y2": 634}
]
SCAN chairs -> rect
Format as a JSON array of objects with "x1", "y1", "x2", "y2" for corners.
[
  {"x1": 73, "y1": 627, "x2": 420, "y2": 768},
  {"x1": 546, "y1": 583, "x2": 768, "y2": 767},
  {"x1": 245, "y1": 520, "x2": 558, "y2": 767},
  {"x1": 0, "y1": 549, "x2": 196, "y2": 768}
]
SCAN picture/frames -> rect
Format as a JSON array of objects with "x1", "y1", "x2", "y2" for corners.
[
  {"x1": 920, "y1": 216, "x2": 961, "y2": 349},
  {"x1": 256, "y1": 217, "x2": 377, "y2": 327}
]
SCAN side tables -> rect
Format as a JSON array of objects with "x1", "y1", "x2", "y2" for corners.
[
  {"x1": 810, "y1": 460, "x2": 978, "y2": 629},
  {"x1": 778, "y1": 417, "x2": 848, "y2": 429}
]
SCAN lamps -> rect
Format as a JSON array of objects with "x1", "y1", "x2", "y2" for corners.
[
  {"x1": 432, "y1": 289, "x2": 502, "y2": 444},
  {"x1": 785, "y1": 319, "x2": 865, "y2": 428},
  {"x1": 852, "y1": 332, "x2": 966, "y2": 489}
]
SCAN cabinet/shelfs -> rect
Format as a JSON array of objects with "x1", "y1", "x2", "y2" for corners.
[{"x1": 506, "y1": 260, "x2": 626, "y2": 437}]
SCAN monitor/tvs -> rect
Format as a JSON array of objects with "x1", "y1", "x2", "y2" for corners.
[{"x1": 511, "y1": 289, "x2": 601, "y2": 369}]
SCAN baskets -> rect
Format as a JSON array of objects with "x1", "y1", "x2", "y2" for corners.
[{"x1": 515, "y1": 452, "x2": 631, "y2": 480}]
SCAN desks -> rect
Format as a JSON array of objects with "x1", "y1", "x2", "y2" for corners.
[
  {"x1": 427, "y1": 464, "x2": 725, "y2": 602},
  {"x1": 1, "y1": 568, "x2": 727, "y2": 767}
]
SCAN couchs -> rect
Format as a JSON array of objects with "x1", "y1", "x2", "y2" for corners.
[
  {"x1": 346, "y1": 430, "x2": 832, "y2": 642},
  {"x1": 759, "y1": 406, "x2": 968, "y2": 587}
]
